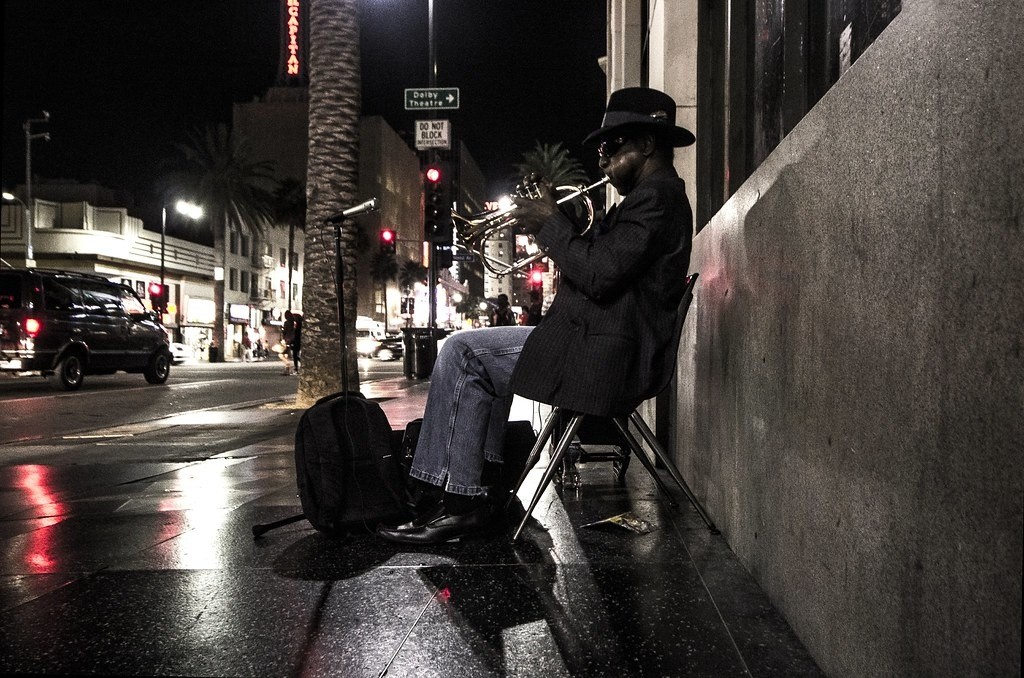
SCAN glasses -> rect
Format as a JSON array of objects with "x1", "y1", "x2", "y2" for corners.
[{"x1": 597, "y1": 128, "x2": 646, "y2": 157}]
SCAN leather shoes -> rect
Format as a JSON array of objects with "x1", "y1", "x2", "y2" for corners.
[{"x1": 377, "y1": 511, "x2": 486, "y2": 547}]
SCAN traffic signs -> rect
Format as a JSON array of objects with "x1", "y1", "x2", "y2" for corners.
[{"x1": 403, "y1": 88, "x2": 460, "y2": 110}]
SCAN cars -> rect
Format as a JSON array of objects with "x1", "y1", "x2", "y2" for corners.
[
  {"x1": 354, "y1": 306, "x2": 523, "y2": 362},
  {"x1": 163, "y1": 325, "x2": 191, "y2": 366}
]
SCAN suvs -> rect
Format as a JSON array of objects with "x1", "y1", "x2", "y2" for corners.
[{"x1": 0, "y1": 266, "x2": 175, "y2": 393}]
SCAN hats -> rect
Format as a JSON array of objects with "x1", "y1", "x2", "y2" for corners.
[{"x1": 581, "y1": 87, "x2": 695, "y2": 150}]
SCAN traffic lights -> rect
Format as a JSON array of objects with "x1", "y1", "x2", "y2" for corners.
[
  {"x1": 531, "y1": 269, "x2": 543, "y2": 294},
  {"x1": 423, "y1": 162, "x2": 454, "y2": 247},
  {"x1": 379, "y1": 229, "x2": 396, "y2": 256},
  {"x1": 148, "y1": 281, "x2": 164, "y2": 306}
]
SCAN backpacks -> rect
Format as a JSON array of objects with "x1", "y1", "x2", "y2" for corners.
[{"x1": 294, "y1": 389, "x2": 400, "y2": 533}]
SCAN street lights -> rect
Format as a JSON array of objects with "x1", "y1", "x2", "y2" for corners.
[
  {"x1": 0, "y1": 191, "x2": 33, "y2": 268},
  {"x1": 160, "y1": 200, "x2": 203, "y2": 324}
]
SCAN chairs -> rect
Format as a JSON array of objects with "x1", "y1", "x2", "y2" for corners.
[{"x1": 503, "y1": 273, "x2": 721, "y2": 548}]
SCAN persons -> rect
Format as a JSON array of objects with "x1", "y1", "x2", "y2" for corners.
[
  {"x1": 375, "y1": 86, "x2": 695, "y2": 547},
  {"x1": 243, "y1": 327, "x2": 270, "y2": 362},
  {"x1": 278, "y1": 310, "x2": 295, "y2": 374},
  {"x1": 290, "y1": 314, "x2": 304, "y2": 375},
  {"x1": 493, "y1": 294, "x2": 515, "y2": 327},
  {"x1": 522, "y1": 290, "x2": 543, "y2": 326}
]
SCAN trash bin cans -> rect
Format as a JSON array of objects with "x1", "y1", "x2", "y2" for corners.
[
  {"x1": 208, "y1": 347, "x2": 218, "y2": 362},
  {"x1": 400, "y1": 328, "x2": 438, "y2": 380}
]
